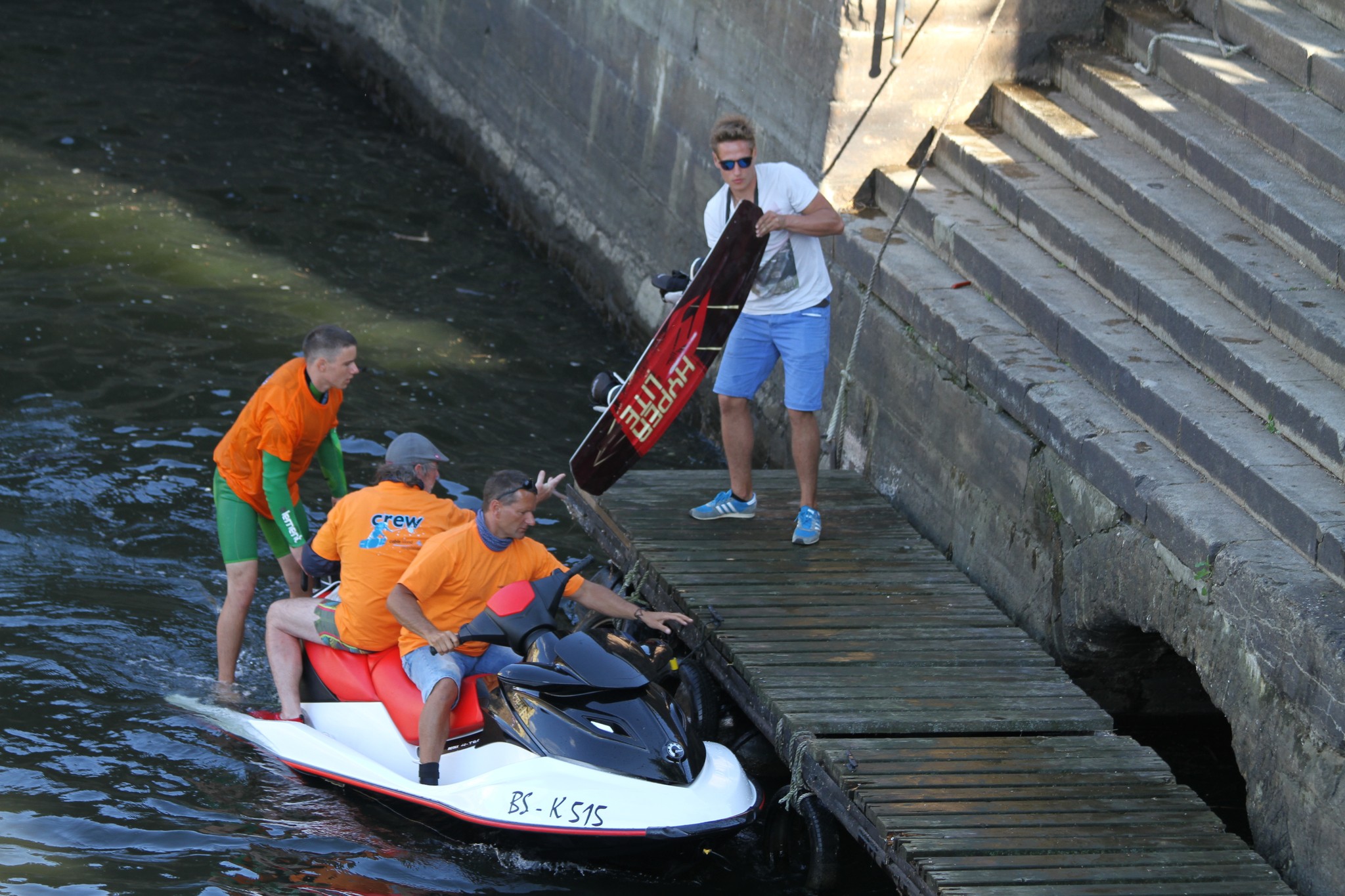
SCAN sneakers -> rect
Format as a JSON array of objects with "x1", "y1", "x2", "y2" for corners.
[
  {"x1": 790, "y1": 506, "x2": 821, "y2": 546},
  {"x1": 689, "y1": 488, "x2": 758, "y2": 520}
]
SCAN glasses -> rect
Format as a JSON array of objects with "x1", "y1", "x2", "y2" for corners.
[
  {"x1": 719, "y1": 156, "x2": 753, "y2": 171},
  {"x1": 495, "y1": 478, "x2": 533, "y2": 500}
]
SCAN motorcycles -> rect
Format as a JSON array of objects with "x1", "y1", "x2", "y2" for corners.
[{"x1": 164, "y1": 507, "x2": 769, "y2": 853}]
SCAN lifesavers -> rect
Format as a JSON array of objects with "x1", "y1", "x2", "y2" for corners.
[
  {"x1": 767, "y1": 784, "x2": 838, "y2": 888},
  {"x1": 574, "y1": 608, "x2": 639, "y2": 638},
  {"x1": 661, "y1": 656, "x2": 722, "y2": 741}
]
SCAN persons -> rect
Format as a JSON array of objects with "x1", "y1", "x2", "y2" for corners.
[
  {"x1": 249, "y1": 431, "x2": 566, "y2": 726},
  {"x1": 687, "y1": 118, "x2": 846, "y2": 544},
  {"x1": 212, "y1": 329, "x2": 360, "y2": 683},
  {"x1": 385, "y1": 470, "x2": 692, "y2": 784}
]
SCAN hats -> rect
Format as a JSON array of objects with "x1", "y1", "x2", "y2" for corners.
[{"x1": 383, "y1": 432, "x2": 450, "y2": 467}]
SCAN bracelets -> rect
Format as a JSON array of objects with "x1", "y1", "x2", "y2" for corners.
[{"x1": 634, "y1": 608, "x2": 646, "y2": 621}]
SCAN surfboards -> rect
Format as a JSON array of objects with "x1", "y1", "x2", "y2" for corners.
[{"x1": 569, "y1": 199, "x2": 771, "y2": 495}]
